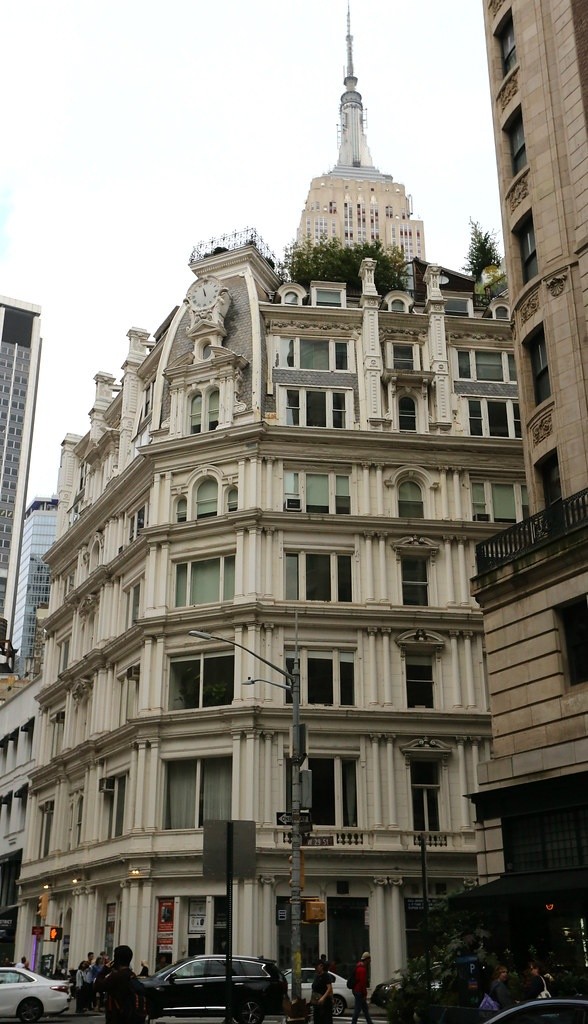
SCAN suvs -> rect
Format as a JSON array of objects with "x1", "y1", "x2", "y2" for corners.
[{"x1": 134, "y1": 954, "x2": 288, "y2": 1024}]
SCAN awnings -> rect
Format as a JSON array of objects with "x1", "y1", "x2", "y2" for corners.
[{"x1": 448, "y1": 869, "x2": 588, "y2": 909}]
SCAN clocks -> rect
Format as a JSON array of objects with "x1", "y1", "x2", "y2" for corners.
[{"x1": 186, "y1": 276, "x2": 221, "y2": 311}]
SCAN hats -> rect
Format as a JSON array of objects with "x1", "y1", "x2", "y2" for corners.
[{"x1": 361, "y1": 951, "x2": 370, "y2": 960}]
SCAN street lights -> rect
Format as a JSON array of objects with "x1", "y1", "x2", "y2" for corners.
[{"x1": 189, "y1": 628, "x2": 301, "y2": 1004}]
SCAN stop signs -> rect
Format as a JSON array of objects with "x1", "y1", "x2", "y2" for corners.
[{"x1": 50, "y1": 927, "x2": 62, "y2": 940}]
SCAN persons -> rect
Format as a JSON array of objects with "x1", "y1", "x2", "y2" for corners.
[
  {"x1": 1, "y1": 956, "x2": 30, "y2": 971},
  {"x1": 75, "y1": 951, "x2": 168, "y2": 1014},
  {"x1": 307, "y1": 954, "x2": 336, "y2": 1024},
  {"x1": 488, "y1": 962, "x2": 559, "y2": 1008},
  {"x1": 351, "y1": 951, "x2": 374, "y2": 1024},
  {"x1": 106, "y1": 945, "x2": 157, "y2": 1024}
]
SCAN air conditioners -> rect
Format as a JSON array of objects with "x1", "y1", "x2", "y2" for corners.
[
  {"x1": 119, "y1": 545, "x2": 128, "y2": 553},
  {"x1": 99, "y1": 778, "x2": 114, "y2": 792},
  {"x1": 44, "y1": 801, "x2": 54, "y2": 814},
  {"x1": 284, "y1": 498, "x2": 302, "y2": 511},
  {"x1": 56, "y1": 712, "x2": 65, "y2": 723},
  {"x1": 127, "y1": 666, "x2": 139, "y2": 681}
]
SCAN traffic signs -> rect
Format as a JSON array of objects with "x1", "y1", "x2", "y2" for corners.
[{"x1": 276, "y1": 812, "x2": 313, "y2": 833}]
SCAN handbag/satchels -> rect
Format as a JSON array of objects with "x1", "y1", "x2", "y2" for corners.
[
  {"x1": 537, "y1": 976, "x2": 552, "y2": 1000},
  {"x1": 310, "y1": 991, "x2": 325, "y2": 1006},
  {"x1": 346, "y1": 968, "x2": 355, "y2": 989}
]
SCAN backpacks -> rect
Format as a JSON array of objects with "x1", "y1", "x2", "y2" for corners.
[{"x1": 97, "y1": 970, "x2": 119, "y2": 1003}]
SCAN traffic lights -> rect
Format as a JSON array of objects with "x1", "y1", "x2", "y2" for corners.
[
  {"x1": 38, "y1": 892, "x2": 48, "y2": 920},
  {"x1": 289, "y1": 850, "x2": 304, "y2": 891}
]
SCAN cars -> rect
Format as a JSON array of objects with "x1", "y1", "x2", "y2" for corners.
[
  {"x1": 277, "y1": 968, "x2": 356, "y2": 1017},
  {"x1": 479, "y1": 997, "x2": 588, "y2": 1024},
  {"x1": 372, "y1": 962, "x2": 448, "y2": 1008},
  {"x1": 0, "y1": 967, "x2": 74, "y2": 1024}
]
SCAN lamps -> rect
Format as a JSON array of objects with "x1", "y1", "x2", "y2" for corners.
[{"x1": 7, "y1": 672, "x2": 39, "y2": 691}]
